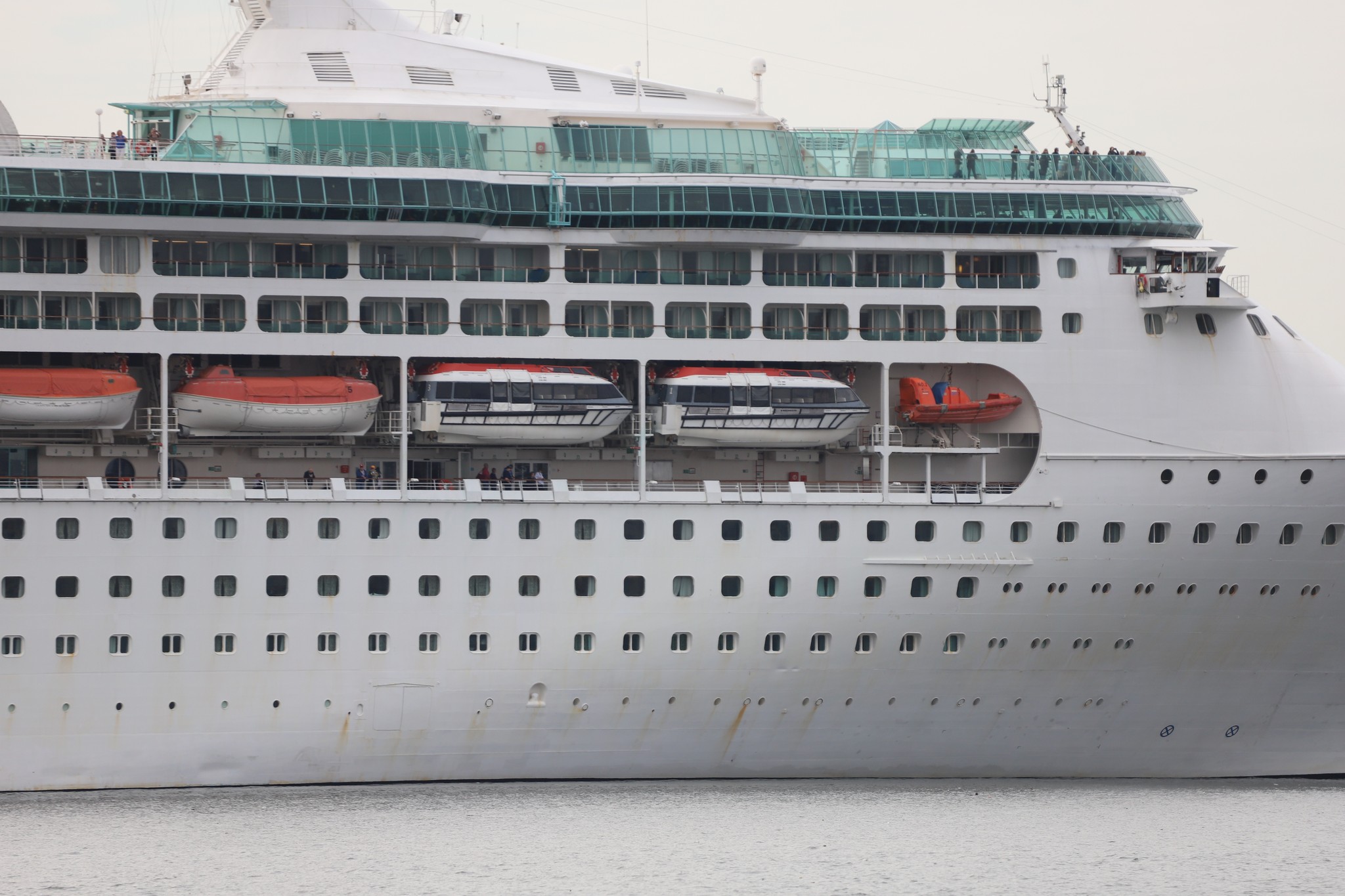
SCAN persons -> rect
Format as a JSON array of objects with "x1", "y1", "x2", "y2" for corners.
[
  {"x1": 953, "y1": 145, "x2": 1146, "y2": 181},
  {"x1": 96, "y1": 126, "x2": 162, "y2": 161},
  {"x1": 253, "y1": 473, "x2": 266, "y2": 489},
  {"x1": 77, "y1": 482, "x2": 88, "y2": 489},
  {"x1": 507, "y1": 464, "x2": 515, "y2": 483},
  {"x1": 534, "y1": 468, "x2": 546, "y2": 490},
  {"x1": 526, "y1": 471, "x2": 537, "y2": 490},
  {"x1": 476, "y1": 463, "x2": 491, "y2": 490},
  {"x1": 368, "y1": 465, "x2": 382, "y2": 489},
  {"x1": 304, "y1": 468, "x2": 315, "y2": 489},
  {"x1": 356, "y1": 464, "x2": 368, "y2": 489},
  {"x1": 376, "y1": 467, "x2": 382, "y2": 490},
  {"x1": 503, "y1": 465, "x2": 514, "y2": 490},
  {"x1": 1172, "y1": 264, "x2": 1182, "y2": 272},
  {"x1": 490, "y1": 468, "x2": 500, "y2": 490}
]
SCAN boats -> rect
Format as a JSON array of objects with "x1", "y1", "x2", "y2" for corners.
[
  {"x1": 168, "y1": 362, "x2": 387, "y2": 447},
  {"x1": 649, "y1": 359, "x2": 875, "y2": 454},
  {"x1": 405, "y1": 352, "x2": 636, "y2": 459},
  {"x1": 0, "y1": 368, "x2": 141, "y2": 432},
  {"x1": 896, "y1": 377, "x2": 1021, "y2": 427}
]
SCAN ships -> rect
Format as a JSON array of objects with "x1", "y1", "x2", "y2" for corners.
[{"x1": 0, "y1": 0, "x2": 1345, "y2": 796}]
